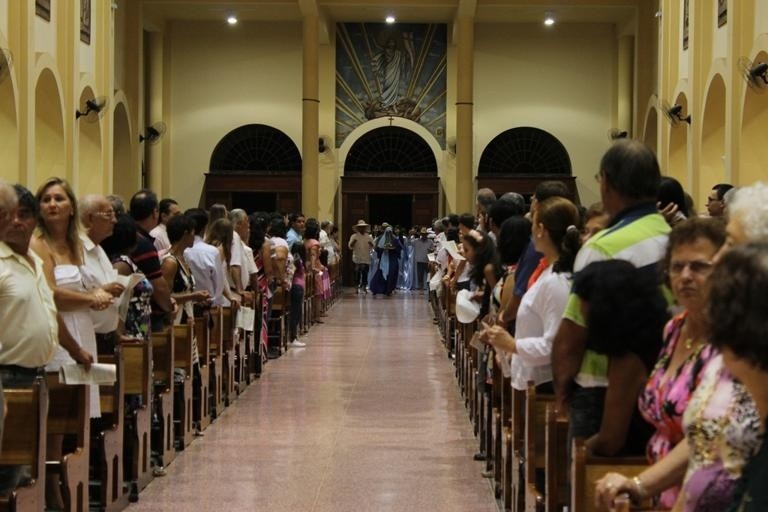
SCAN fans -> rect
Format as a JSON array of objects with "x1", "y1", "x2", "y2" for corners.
[
  {"x1": 737, "y1": 56, "x2": 768, "y2": 96},
  {"x1": 317, "y1": 135, "x2": 335, "y2": 157},
  {"x1": 140, "y1": 122, "x2": 166, "y2": 145},
  {"x1": 607, "y1": 129, "x2": 627, "y2": 144},
  {"x1": 447, "y1": 138, "x2": 457, "y2": 159},
  {"x1": 649, "y1": 94, "x2": 691, "y2": 129},
  {"x1": 76, "y1": 95, "x2": 111, "y2": 125}
]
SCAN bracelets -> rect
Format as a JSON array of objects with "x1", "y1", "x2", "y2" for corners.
[{"x1": 634, "y1": 476, "x2": 649, "y2": 503}]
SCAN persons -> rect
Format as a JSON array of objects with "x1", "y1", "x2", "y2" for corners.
[
  {"x1": 433, "y1": 189, "x2": 503, "y2": 323},
  {"x1": 28, "y1": 176, "x2": 115, "y2": 465},
  {"x1": 500, "y1": 192, "x2": 526, "y2": 217},
  {"x1": 203, "y1": 203, "x2": 227, "y2": 239},
  {"x1": 247, "y1": 208, "x2": 343, "y2": 351},
  {"x1": 161, "y1": 214, "x2": 215, "y2": 384},
  {"x1": 127, "y1": 189, "x2": 178, "y2": 339},
  {"x1": 703, "y1": 242, "x2": 768, "y2": 512},
  {"x1": 550, "y1": 136, "x2": 664, "y2": 461},
  {"x1": 347, "y1": 219, "x2": 433, "y2": 298},
  {"x1": 479, "y1": 197, "x2": 581, "y2": 489},
  {"x1": 77, "y1": 193, "x2": 127, "y2": 357},
  {"x1": 565, "y1": 259, "x2": 661, "y2": 460},
  {"x1": 205, "y1": 218, "x2": 241, "y2": 312},
  {"x1": 105, "y1": 195, "x2": 127, "y2": 220},
  {"x1": 149, "y1": 198, "x2": 181, "y2": 263},
  {"x1": 704, "y1": 182, "x2": 734, "y2": 217},
  {"x1": 513, "y1": 180, "x2": 573, "y2": 312},
  {"x1": 185, "y1": 207, "x2": 223, "y2": 311},
  {"x1": 594, "y1": 215, "x2": 725, "y2": 512},
  {"x1": 655, "y1": 176, "x2": 685, "y2": 225},
  {"x1": 672, "y1": 180, "x2": 768, "y2": 512},
  {"x1": 102, "y1": 216, "x2": 167, "y2": 478},
  {"x1": 500, "y1": 217, "x2": 532, "y2": 323},
  {"x1": 584, "y1": 203, "x2": 612, "y2": 243},
  {"x1": 0, "y1": 183, "x2": 93, "y2": 512},
  {"x1": 228, "y1": 208, "x2": 252, "y2": 304}
]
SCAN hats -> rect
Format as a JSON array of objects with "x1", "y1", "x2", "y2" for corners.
[{"x1": 352, "y1": 220, "x2": 371, "y2": 232}]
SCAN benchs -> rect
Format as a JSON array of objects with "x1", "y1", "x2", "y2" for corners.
[
  {"x1": 266, "y1": 261, "x2": 343, "y2": 360},
  {"x1": 472, "y1": 352, "x2": 657, "y2": 511},
  {"x1": 0, "y1": 334, "x2": 155, "y2": 512},
  {"x1": 426, "y1": 261, "x2": 466, "y2": 353},
  {"x1": 151, "y1": 290, "x2": 266, "y2": 469},
  {"x1": 453, "y1": 316, "x2": 497, "y2": 437}
]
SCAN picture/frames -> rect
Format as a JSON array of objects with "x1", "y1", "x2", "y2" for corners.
[{"x1": 80, "y1": 1, "x2": 92, "y2": 45}]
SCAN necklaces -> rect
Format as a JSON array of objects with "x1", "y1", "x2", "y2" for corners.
[{"x1": 680, "y1": 323, "x2": 706, "y2": 353}]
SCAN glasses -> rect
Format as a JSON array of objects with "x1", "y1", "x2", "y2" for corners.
[{"x1": 596, "y1": 170, "x2": 604, "y2": 181}]
